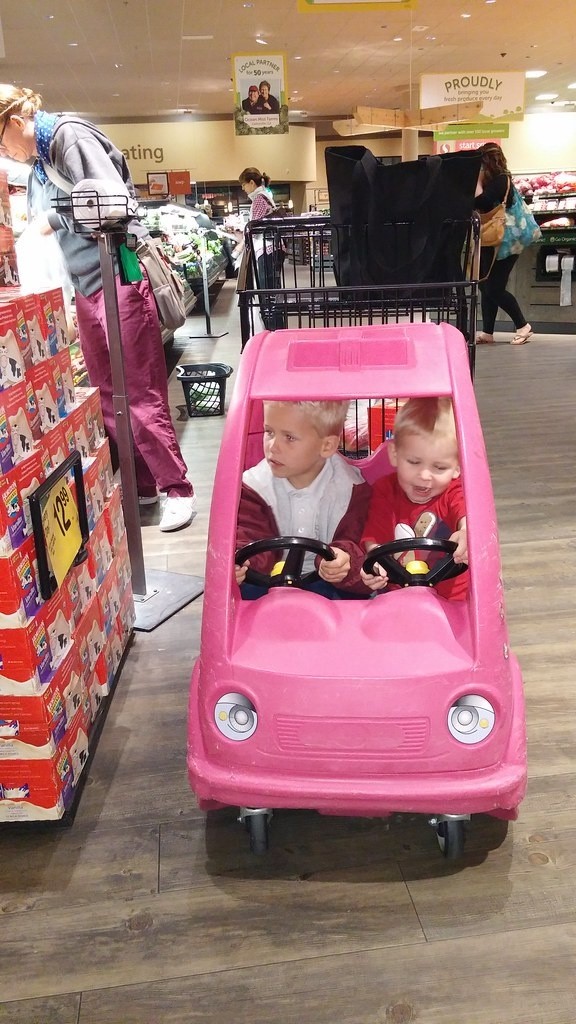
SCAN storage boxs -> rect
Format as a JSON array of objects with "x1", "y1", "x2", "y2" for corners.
[{"x1": 0, "y1": 170, "x2": 137, "y2": 823}]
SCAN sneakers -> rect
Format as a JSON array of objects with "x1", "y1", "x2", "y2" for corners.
[
  {"x1": 159, "y1": 495, "x2": 195, "y2": 531},
  {"x1": 120, "y1": 495, "x2": 157, "y2": 505}
]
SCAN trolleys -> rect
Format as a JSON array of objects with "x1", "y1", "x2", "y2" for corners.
[{"x1": 234, "y1": 204, "x2": 482, "y2": 378}]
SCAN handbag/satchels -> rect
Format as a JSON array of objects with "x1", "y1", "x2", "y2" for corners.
[
  {"x1": 476, "y1": 203, "x2": 506, "y2": 246},
  {"x1": 339, "y1": 399, "x2": 397, "y2": 452},
  {"x1": 257, "y1": 193, "x2": 293, "y2": 227},
  {"x1": 493, "y1": 182, "x2": 542, "y2": 260},
  {"x1": 325, "y1": 145, "x2": 484, "y2": 308},
  {"x1": 135, "y1": 238, "x2": 187, "y2": 329}
]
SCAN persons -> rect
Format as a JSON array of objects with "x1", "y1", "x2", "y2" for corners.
[
  {"x1": 233, "y1": 399, "x2": 372, "y2": 602},
  {"x1": 358, "y1": 396, "x2": 472, "y2": 605},
  {"x1": 242, "y1": 80, "x2": 280, "y2": 115},
  {"x1": 0, "y1": 84, "x2": 197, "y2": 530},
  {"x1": 473, "y1": 142, "x2": 534, "y2": 345},
  {"x1": 227, "y1": 168, "x2": 286, "y2": 332}
]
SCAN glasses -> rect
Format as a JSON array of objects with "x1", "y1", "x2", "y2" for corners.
[
  {"x1": 242, "y1": 181, "x2": 248, "y2": 188},
  {"x1": 0, "y1": 113, "x2": 8, "y2": 149}
]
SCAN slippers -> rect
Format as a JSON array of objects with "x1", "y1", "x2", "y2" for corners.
[
  {"x1": 511, "y1": 332, "x2": 533, "y2": 344},
  {"x1": 476, "y1": 336, "x2": 495, "y2": 344}
]
